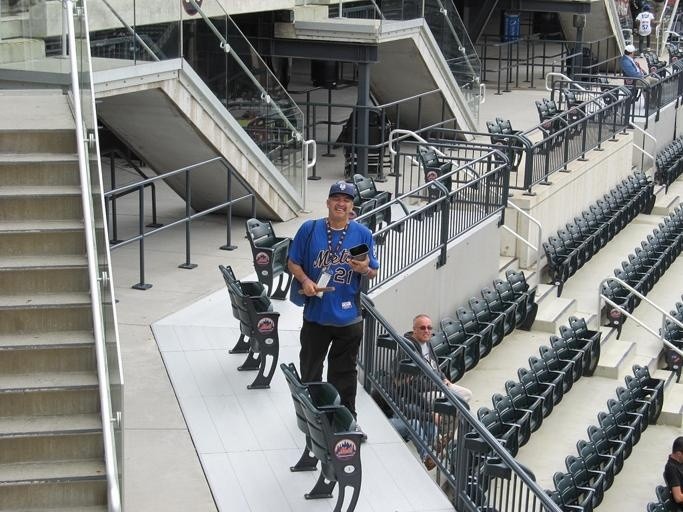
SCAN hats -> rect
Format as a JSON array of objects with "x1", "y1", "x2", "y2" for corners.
[
  {"x1": 625, "y1": 45, "x2": 636, "y2": 53},
  {"x1": 329, "y1": 181, "x2": 357, "y2": 200}
]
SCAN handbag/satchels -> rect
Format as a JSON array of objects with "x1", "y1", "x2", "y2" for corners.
[{"x1": 290, "y1": 278, "x2": 306, "y2": 307}]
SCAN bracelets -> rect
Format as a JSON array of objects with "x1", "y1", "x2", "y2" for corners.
[
  {"x1": 359, "y1": 267, "x2": 371, "y2": 277},
  {"x1": 300, "y1": 277, "x2": 310, "y2": 284}
]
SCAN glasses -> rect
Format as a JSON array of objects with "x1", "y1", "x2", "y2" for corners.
[{"x1": 416, "y1": 326, "x2": 432, "y2": 330}]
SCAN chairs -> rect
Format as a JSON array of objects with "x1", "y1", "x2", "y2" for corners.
[
  {"x1": 246, "y1": 42, "x2": 683, "y2": 511},
  {"x1": 217, "y1": 262, "x2": 269, "y2": 373},
  {"x1": 228, "y1": 286, "x2": 281, "y2": 390}
]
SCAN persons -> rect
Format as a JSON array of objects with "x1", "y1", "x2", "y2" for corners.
[
  {"x1": 628, "y1": 0, "x2": 641, "y2": 36},
  {"x1": 634, "y1": 4, "x2": 660, "y2": 58},
  {"x1": 287, "y1": 180, "x2": 378, "y2": 442},
  {"x1": 664, "y1": 437, "x2": 683, "y2": 511},
  {"x1": 391, "y1": 314, "x2": 472, "y2": 441},
  {"x1": 367, "y1": 369, "x2": 455, "y2": 471},
  {"x1": 619, "y1": 44, "x2": 666, "y2": 109}
]
